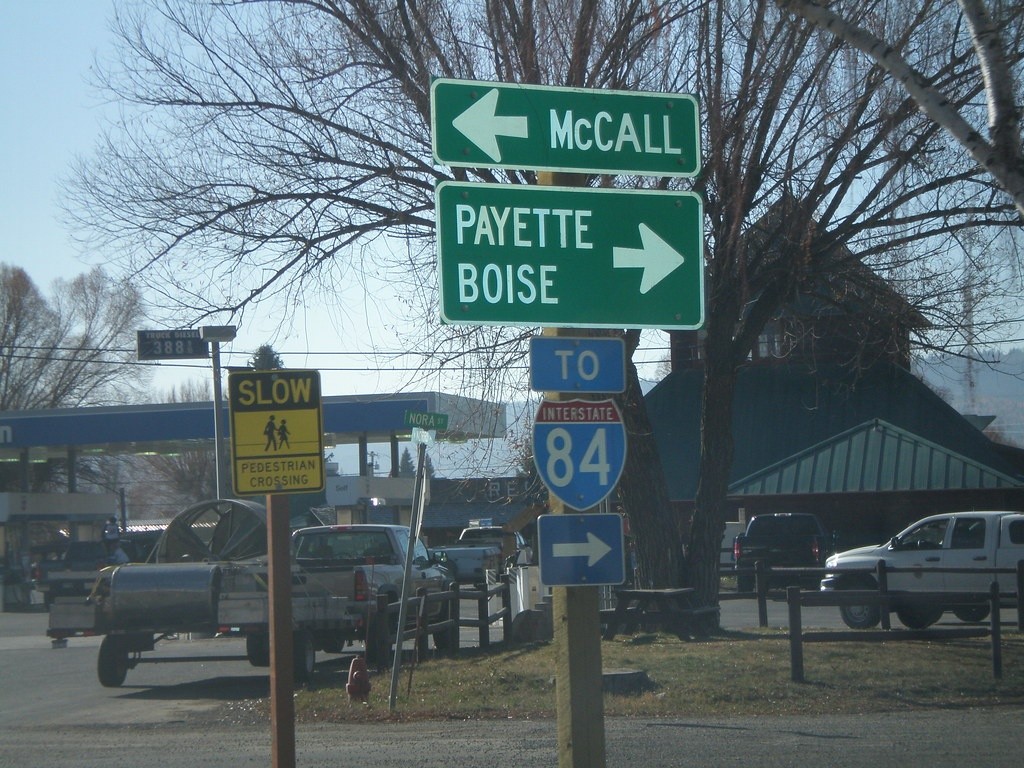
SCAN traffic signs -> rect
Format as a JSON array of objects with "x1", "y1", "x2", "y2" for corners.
[
  {"x1": 429, "y1": 79, "x2": 702, "y2": 179},
  {"x1": 434, "y1": 180, "x2": 706, "y2": 332}
]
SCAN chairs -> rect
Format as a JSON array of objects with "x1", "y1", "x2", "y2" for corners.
[
  {"x1": 953, "y1": 524, "x2": 976, "y2": 550},
  {"x1": 315, "y1": 544, "x2": 335, "y2": 560}
]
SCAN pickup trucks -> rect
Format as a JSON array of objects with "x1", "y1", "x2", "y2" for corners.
[
  {"x1": 818, "y1": 511, "x2": 1024, "y2": 629},
  {"x1": 218, "y1": 524, "x2": 460, "y2": 677},
  {"x1": 95, "y1": 496, "x2": 295, "y2": 691},
  {"x1": 734, "y1": 512, "x2": 833, "y2": 593},
  {"x1": 426, "y1": 525, "x2": 529, "y2": 588}
]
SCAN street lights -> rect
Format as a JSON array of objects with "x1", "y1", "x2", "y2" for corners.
[{"x1": 198, "y1": 325, "x2": 237, "y2": 524}]
